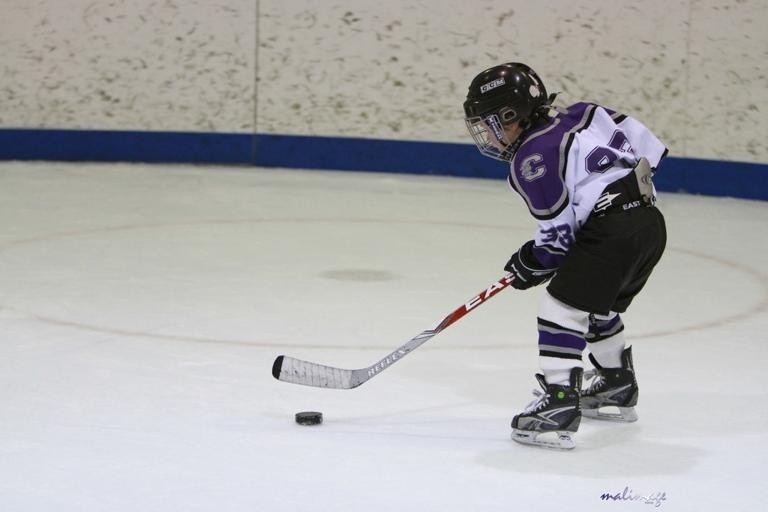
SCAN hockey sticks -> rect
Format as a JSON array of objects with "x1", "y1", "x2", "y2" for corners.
[{"x1": 271, "y1": 272, "x2": 516, "y2": 390}]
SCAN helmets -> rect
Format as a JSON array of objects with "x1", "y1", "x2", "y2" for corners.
[{"x1": 463, "y1": 62, "x2": 547, "y2": 164}]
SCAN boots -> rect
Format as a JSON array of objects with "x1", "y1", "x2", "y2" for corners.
[
  {"x1": 510, "y1": 366, "x2": 582, "y2": 433},
  {"x1": 579, "y1": 346, "x2": 638, "y2": 408}
]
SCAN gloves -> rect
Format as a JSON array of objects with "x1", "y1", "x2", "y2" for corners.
[{"x1": 504, "y1": 239, "x2": 555, "y2": 291}]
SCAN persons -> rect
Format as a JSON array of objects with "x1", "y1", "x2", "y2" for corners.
[{"x1": 462, "y1": 61, "x2": 668, "y2": 452}]
